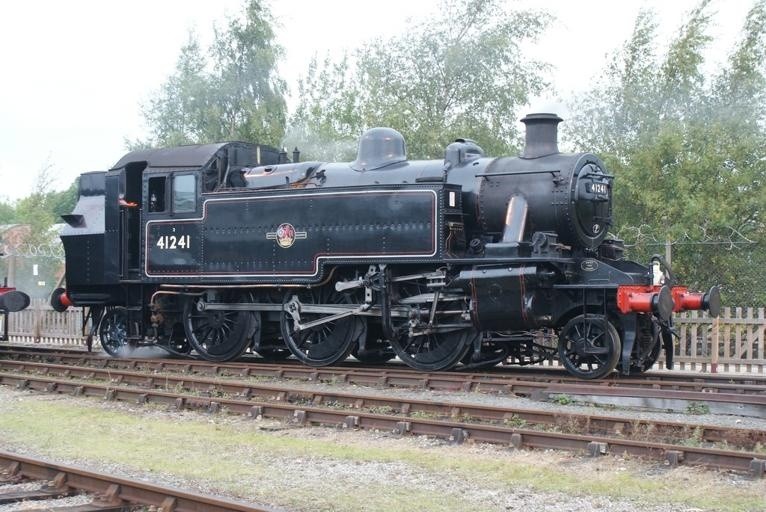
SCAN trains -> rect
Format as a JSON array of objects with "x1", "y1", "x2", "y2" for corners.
[{"x1": 0, "y1": 111, "x2": 725, "y2": 381}]
